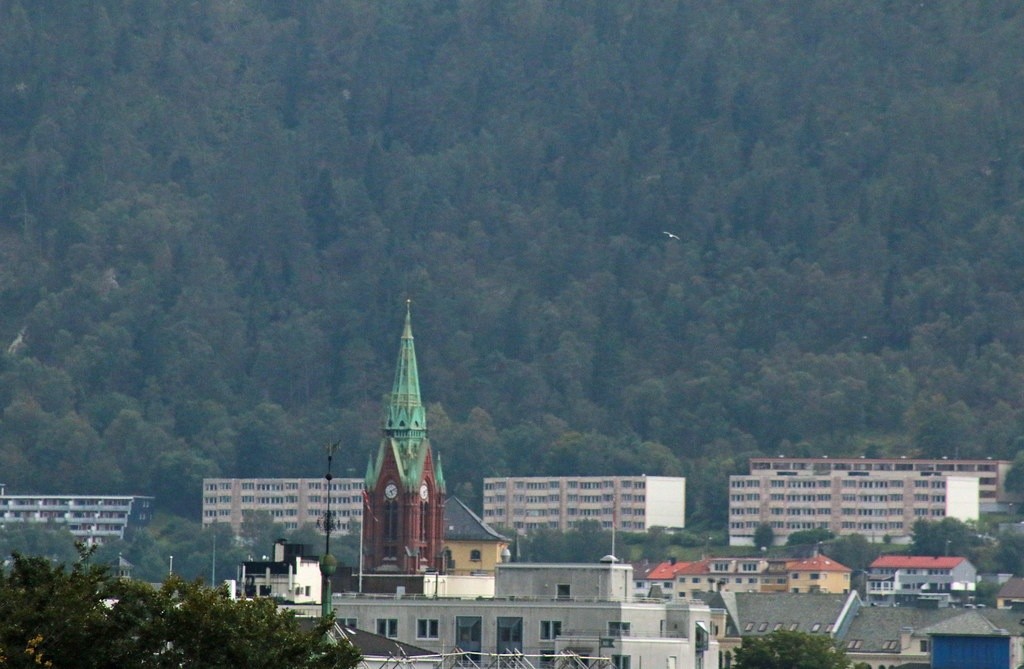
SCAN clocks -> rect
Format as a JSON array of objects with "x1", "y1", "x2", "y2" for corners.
[
  {"x1": 384, "y1": 483, "x2": 399, "y2": 500},
  {"x1": 420, "y1": 484, "x2": 429, "y2": 500}
]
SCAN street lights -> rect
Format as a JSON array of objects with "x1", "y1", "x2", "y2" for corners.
[
  {"x1": 212, "y1": 534, "x2": 215, "y2": 586},
  {"x1": 170, "y1": 555, "x2": 173, "y2": 574}
]
